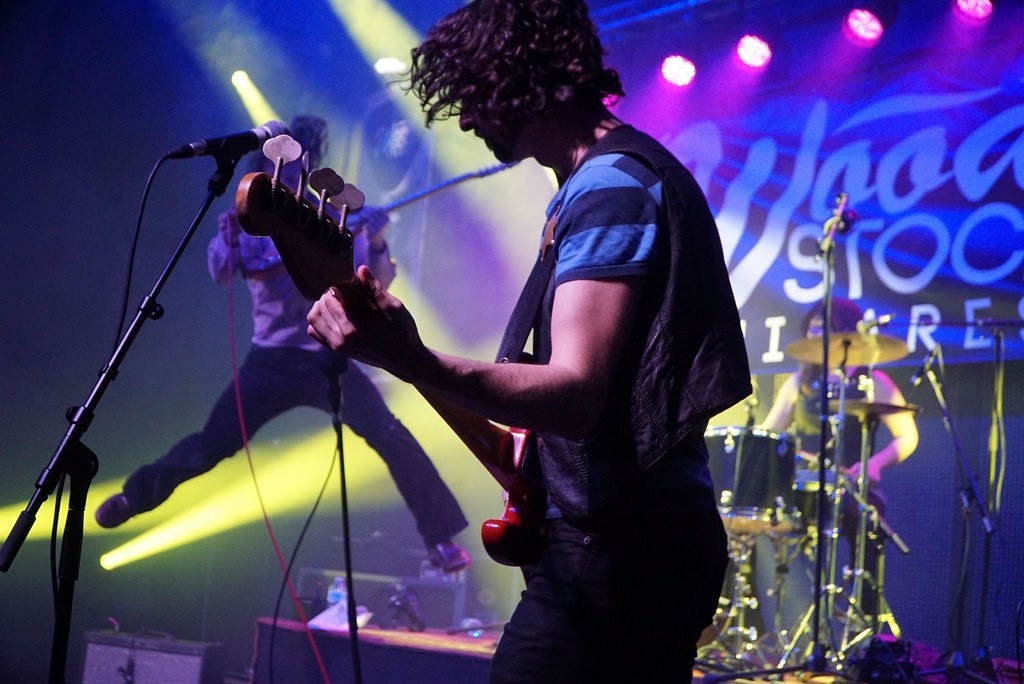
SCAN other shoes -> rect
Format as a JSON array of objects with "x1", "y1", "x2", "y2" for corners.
[
  {"x1": 94, "y1": 493, "x2": 134, "y2": 528},
  {"x1": 428, "y1": 537, "x2": 471, "y2": 571}
]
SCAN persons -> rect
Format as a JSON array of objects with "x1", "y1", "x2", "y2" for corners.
[
  {"x1": 303, "y1": 0, "x2": 756, "y2": 684},
  {"x1": 749, "y1": 302, "x2": 926, "y2": 647},
  {"x1": 95, "y1": 118, "x2": 475, "y2": 572}
]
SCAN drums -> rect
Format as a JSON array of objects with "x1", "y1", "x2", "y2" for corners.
[
  {"x1": 795, "y1": 481, "x2": 842, "y2": 536},
  {"x1": 703, "y1": 427, "x2": 795, "y2": 520}
]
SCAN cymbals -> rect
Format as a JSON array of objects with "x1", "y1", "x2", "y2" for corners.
[
  {"x1": 828, "y1": 399, "x2": 922, "y2": 418},
  {"x1": 785, "y1": 331, "x2": 916, "y2": 368}
]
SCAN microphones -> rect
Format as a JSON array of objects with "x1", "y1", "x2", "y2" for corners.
[
  {"x1": 855, "y1": 311, "x2": 895, "y2": 334},
  {"x1": 909, "y1": 347, "x2": 938, "y2": 386},
  {"x1": 168, "y1": 120, "x2": 295, "y2": 164}
]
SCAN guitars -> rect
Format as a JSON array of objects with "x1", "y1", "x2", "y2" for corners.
[{"x1": 234, "y1": 134, "x2": 555, "y2": 585}]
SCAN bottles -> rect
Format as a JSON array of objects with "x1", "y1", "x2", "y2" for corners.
[
  {"x1": 327, "y1": 578, "x2": 347, "y2": 607},
  {"x1": 460, "y1": 618, "x2": 482, "y2": 638}
]
